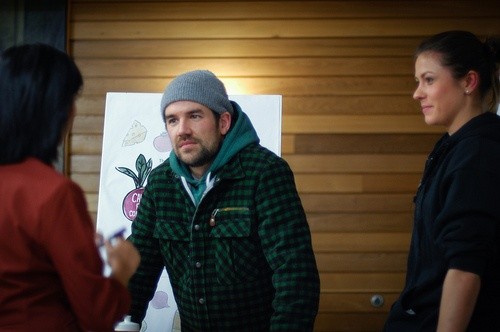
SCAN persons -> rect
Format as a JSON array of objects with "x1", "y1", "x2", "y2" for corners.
[
  {"x1": 0, "y1": 44, "x2": 140, "y2": 332},
  {"x1": 113, "y1": 68, "x2": 320, "y2": 331},
  {"x1": 382, "y1": 31, "x2": 500, "y2": 332}
]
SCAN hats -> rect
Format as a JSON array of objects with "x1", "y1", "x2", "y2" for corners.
[{"x1": 161, "y1": 70, "x2": 228, "y2": 129}]
